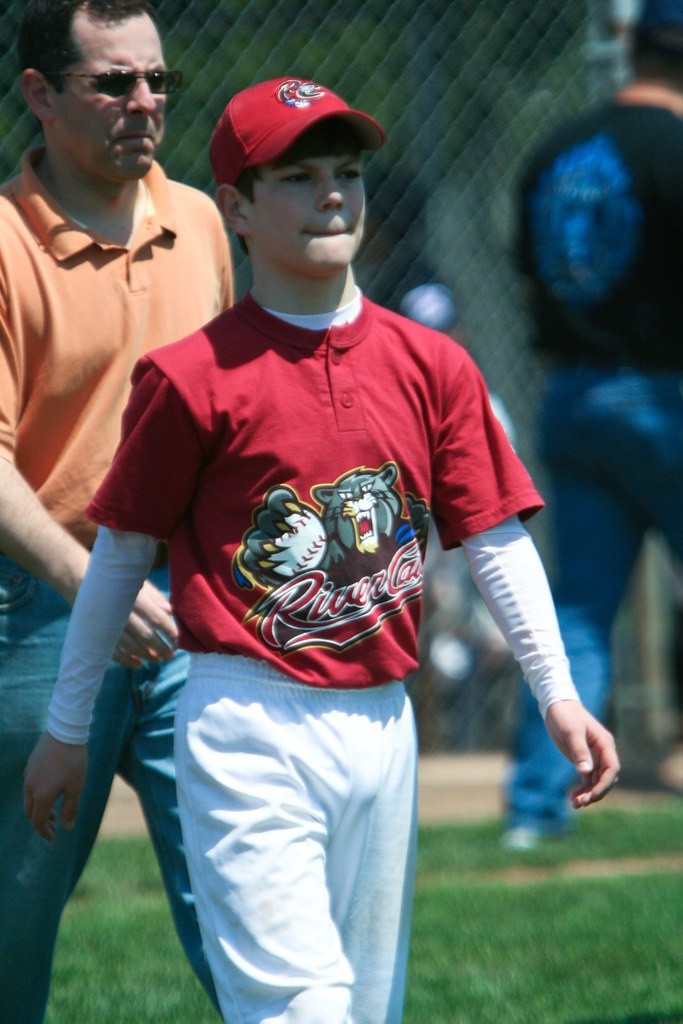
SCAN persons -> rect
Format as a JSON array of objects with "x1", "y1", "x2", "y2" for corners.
[
  {"x1": 492, "y1": 0, "x2": 683, "y2": 854},
  {"x1": 0, "y1": 0, "x2": 234, "y2": 1024},
  {"x1": 359, "y1": 157, "x2": 518, "y2": 753},
  {"x1": 18, "y1": 79, "x2": 622, "y2": 1024}
]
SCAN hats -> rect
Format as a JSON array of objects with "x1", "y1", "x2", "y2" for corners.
[{"x1": 210, "y1": 77, "x2": 385, "y2": 186}]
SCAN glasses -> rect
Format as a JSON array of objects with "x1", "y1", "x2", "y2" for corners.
[{"x1": 45, "y1": 70, "x2": 182, "y2": 96}]
